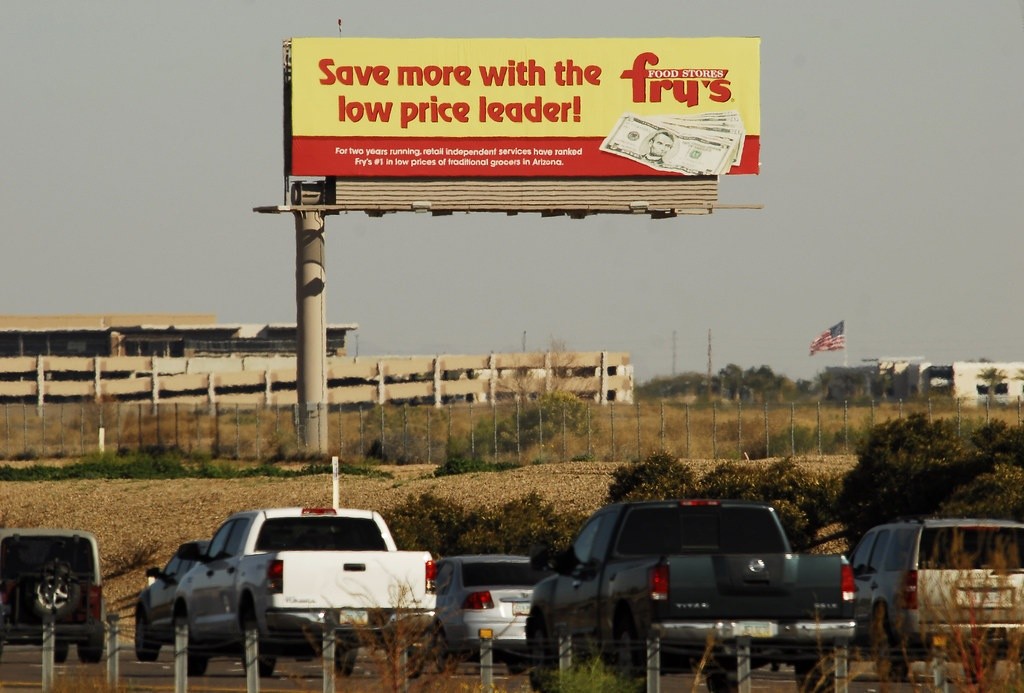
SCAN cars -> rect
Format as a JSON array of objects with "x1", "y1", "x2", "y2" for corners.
[
  {"x1": 132, "y1": 538, "x2": 212, "y2": 661},
  {"x1": 432, "y1": 554, "x2": 535, "y2": 675}
]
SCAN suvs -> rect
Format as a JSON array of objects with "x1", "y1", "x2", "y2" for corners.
[
  {"x1": 0, "y1": 527, "x2": 107, "y2": 663},
  {"x1": 849, "y1": 518, "x2": 1024, "y2": 678}
]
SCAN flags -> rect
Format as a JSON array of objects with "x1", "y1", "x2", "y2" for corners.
[{"x1": 809, "y1": 320, "x2": 847, "y2": 356}]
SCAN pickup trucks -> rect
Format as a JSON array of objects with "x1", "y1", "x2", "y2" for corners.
[
  {"x1": 172, "y1": 506, "x2": 439, "y2": 678},
  {"x1": 524, "y1": 500, "x2": 856, "y2": 693}
]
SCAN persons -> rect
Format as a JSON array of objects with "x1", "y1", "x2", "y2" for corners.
[{"x1": 640, "y1": 132, "x2": 674, "y2": 167}]
